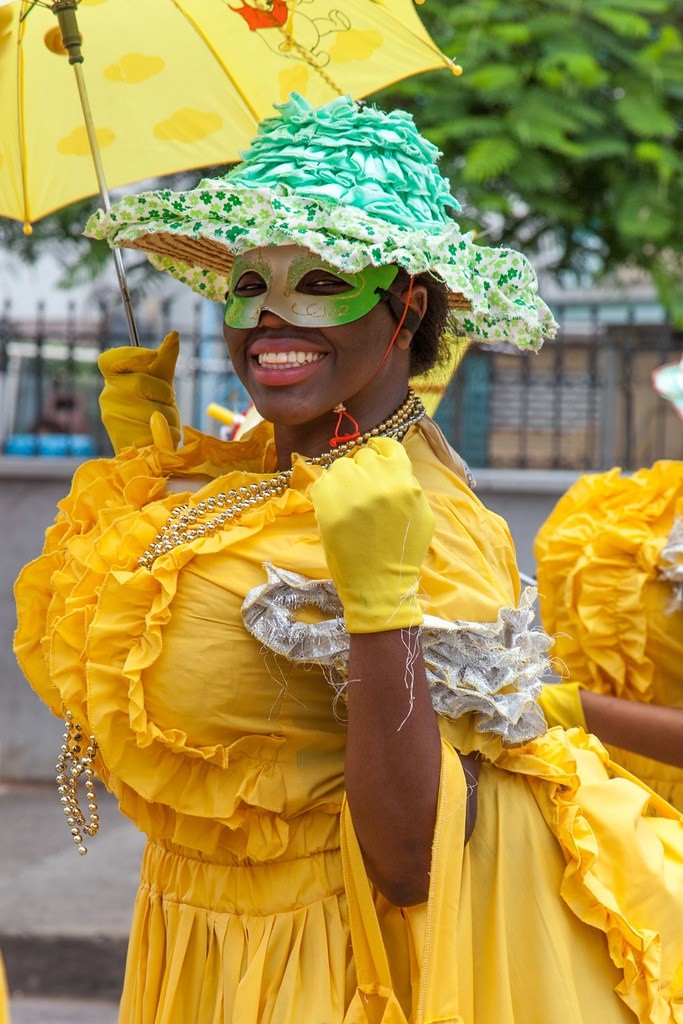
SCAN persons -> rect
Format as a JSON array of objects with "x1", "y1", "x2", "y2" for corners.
[
  {"x1": 14, "y1": 90, "x2": 683, "y2": 1024},
  {"x1": 534, "y1": 359, "x2": 682, "y2": 814}
]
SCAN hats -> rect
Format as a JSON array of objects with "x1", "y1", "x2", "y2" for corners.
[{"x1": 90, "y1": 94, "x2": 558, "y2": 360}]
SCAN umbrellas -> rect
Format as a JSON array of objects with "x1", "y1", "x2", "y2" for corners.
[{"x1": 0, "y1": 0, "x2": 465, "y2": 451}]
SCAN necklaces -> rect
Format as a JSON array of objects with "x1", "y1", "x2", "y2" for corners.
[{"x1": 55, "y1": 388, "x2": 427, "y2": 858}]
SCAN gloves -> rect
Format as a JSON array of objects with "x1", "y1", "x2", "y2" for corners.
[
  {"x1": 306, "y1": 436, "x2": 424, "y2": 636},
  {"x1": 97, "y1": 331, "x2": 182, "y2": 456}
]
